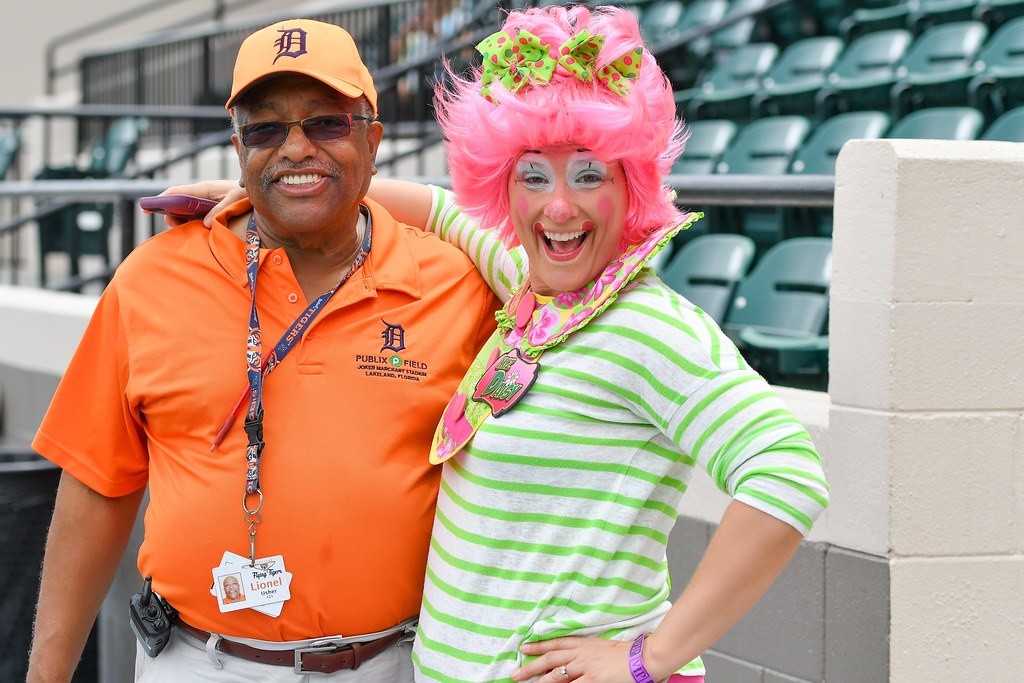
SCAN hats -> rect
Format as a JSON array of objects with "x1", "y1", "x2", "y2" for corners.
[{"x1": 225, "y1": 17, "x2": 380, "y2": 119}]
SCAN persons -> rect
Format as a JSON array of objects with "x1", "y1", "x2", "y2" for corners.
[
  {"x1": 24, "y1": 17, "x2": 500, "y2": 683},
  {"x1": 157, "y1": 7, "x2": 834, "y2": 683}
]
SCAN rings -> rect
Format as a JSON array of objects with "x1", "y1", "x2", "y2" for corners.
[{"x1": 560, "y1": 664, "x2": 572, "y2": 681}]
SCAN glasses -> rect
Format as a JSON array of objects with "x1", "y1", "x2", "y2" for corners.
[{"x1": 234, "y1": 113, "x2": 373, "y2": 150}]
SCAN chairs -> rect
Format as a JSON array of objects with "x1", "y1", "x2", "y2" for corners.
[
  {"x1": 34, "y1": 116, "x2": 151, "y2": 288},
  {"x1": 588, "y1": 0, "x2": 1024, "y2": 375}
]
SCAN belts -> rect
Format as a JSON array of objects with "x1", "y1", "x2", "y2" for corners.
[{"x1": 175, "y1": 618, "x2": 403, "y2": 675}]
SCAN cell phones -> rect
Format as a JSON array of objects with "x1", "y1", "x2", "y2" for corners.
[{"x1": 139, "y1": 194, "x2": 219, "y2": 218}]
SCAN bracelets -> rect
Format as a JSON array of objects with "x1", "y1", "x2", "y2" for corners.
[{"x1": 629, "y1": 632, "x2": 656, "y2": 683}]
